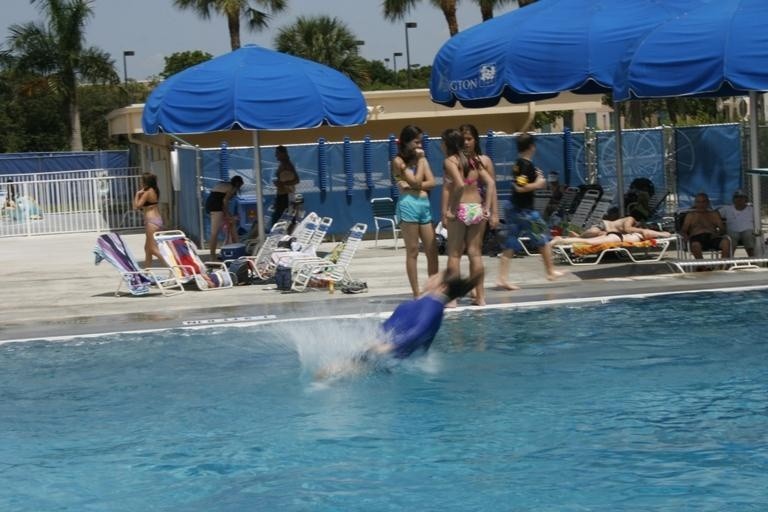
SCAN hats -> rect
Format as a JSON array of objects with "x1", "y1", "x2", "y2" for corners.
[
  {"x1": 733, "y1": 189, "x2": 748, "y2": 199},
  {"x1": 547, "y1": 174, "x2": 559, "y2": 184}
]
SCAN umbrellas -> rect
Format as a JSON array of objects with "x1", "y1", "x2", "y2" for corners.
[{"x1": 427, "y1": 0, "x2": 766, "y2": 217}]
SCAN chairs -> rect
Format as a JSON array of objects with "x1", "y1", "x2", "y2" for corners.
[
  {"x1": 210, "y1": 203, "x2": 368, "y2": 294},
  {"x1": 368, "y1": 194, "x2": 405, "y2": 250},
  {"x1": 89, "y1": 230, "x2": 185, "y2": 299},
  {"x1": 150, "y1": 227, "x2": 234, "y2": 291},
  {"x1": 490, "y1": 183, "x2": 765, "y2": 279}
]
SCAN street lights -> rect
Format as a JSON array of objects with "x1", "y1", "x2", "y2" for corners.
[
  {"x1": 355, "y1": 22, "x2": 420, "y2": 90},
  {"x1": 122, "y1": 50, "x2": 136, "y2": 90}
]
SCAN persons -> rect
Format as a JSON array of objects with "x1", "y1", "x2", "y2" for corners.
[
  {"x1": 204, "y1": 174, "x2": 244, "y2": 260},
  {"x1": 133, "y1": 170, "x2": 168, "y2": 273},
  {"x1": 307, "y1": 268, "x2": 488, "y2": 386},
  {"x1": 680, "y1": 193, "x2": 730, "y2": 271},
  {"x1": 142, "y1": 42, "x2": 368, "y2": 241},
  {"x1": 270, "y1": 146, "x2": 298, "y2": 232},
  {"x1": 570, "y1": 210, "x2": 645, "y2": 239},
  {"x1": 546, "y1": 229, "x2": 672, "y2": 250},
  {"x1": 715, "y1": 189, "x2": 758, "y2": 266},
  {"x1": 391, "y1": 125, "x2": 438, "y2": 302},
  {"x1": 495, "y1": 132, "x2": 570, "y2": 291}
]
